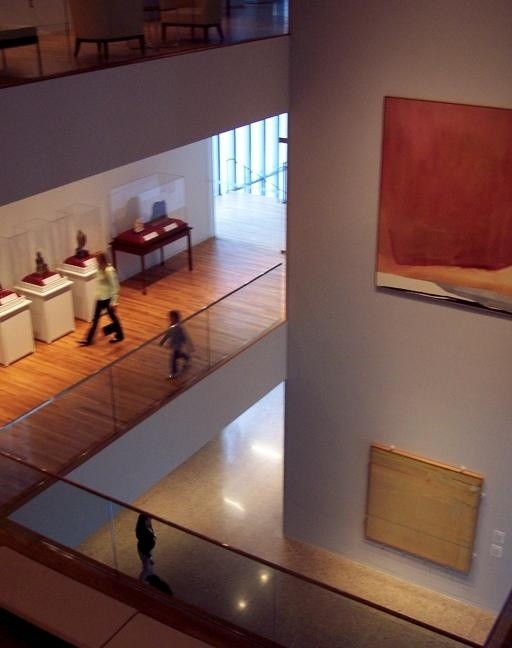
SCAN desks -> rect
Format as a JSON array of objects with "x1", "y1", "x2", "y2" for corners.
[
  {"x1": 161, "y1": 18, "x2": 226, "y2": 44},
  {"x1": 106, "y1": 227, "x2": 194, "y2": 296},
  {"x1": 74, "y1": 32, "x2": 147, "y2": 58}
]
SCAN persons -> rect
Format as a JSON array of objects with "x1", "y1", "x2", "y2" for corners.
[
  {"x1": 135, "y1": 513, "x2": 157, "y2": 576},
  {"x1": 77, "y1": 252, "x2": 125, "y2": 344},
  {"x1": 159, "y1": 309, "x2": 196, "y2": 380}
]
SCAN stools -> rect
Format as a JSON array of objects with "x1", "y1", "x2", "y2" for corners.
[{"x1": 0, "y1": 24, "x2": 44, "y2": 75}]
